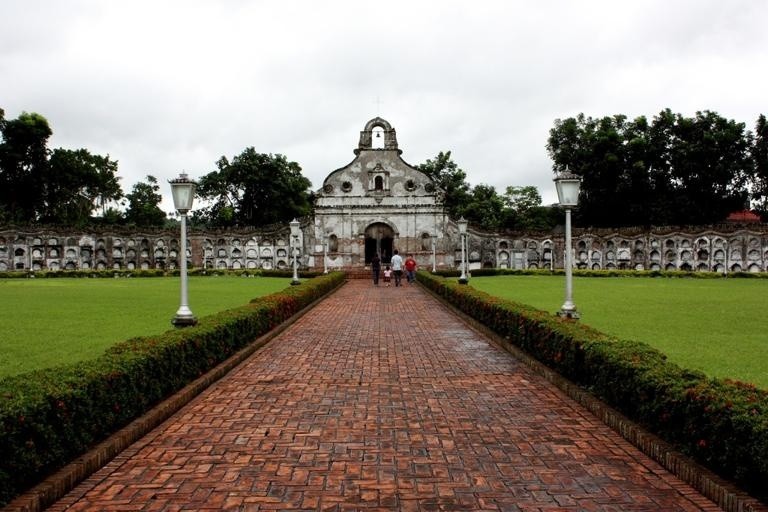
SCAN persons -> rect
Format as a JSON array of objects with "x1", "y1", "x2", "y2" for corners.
[
  {"x1": 371, "y1": 254, "x2": 380, "y2": 285},
  {"x1": 382, "y1": 266, "x2": 391, "y2": 287},
  {"x1": 390, "y1": 249, "x2": 405, "y2": 287},
  {"x1": 405, "y1": 254, "x2": 417, "y2": 284}
]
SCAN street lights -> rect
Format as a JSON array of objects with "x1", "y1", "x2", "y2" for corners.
[
  {"x1": 288, "y1": 218, "x2": 301, "y2": 286},
  {"x1": 552, "y1": 163, "x2": 584, "y2": 318},
  {"x1": 429, "y1": 233, "x2": 438, "y2": 275},
  {"x1": 322, "y1": 232, "x2": 329, "y2": 276},
  {"x1": 166, "y1": 168, "x2": 198, "y2": 329},
  {"x1": 455, "y1": 215, "x2": 469, "y2": 285}
]
